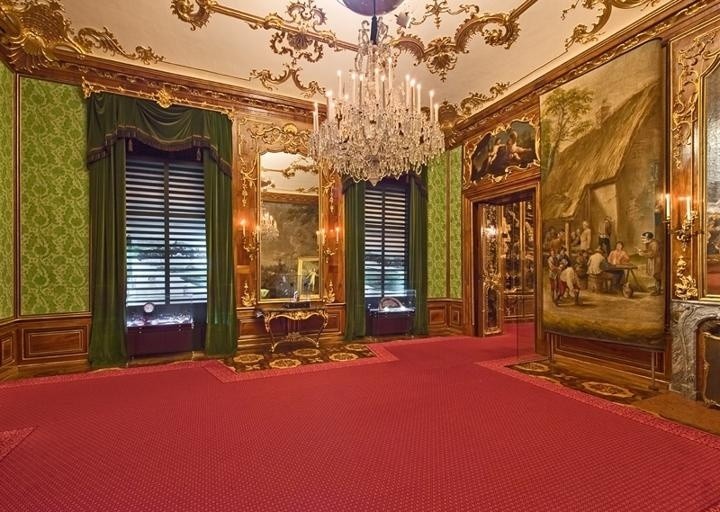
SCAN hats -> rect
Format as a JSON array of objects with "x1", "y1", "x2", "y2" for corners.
[
  {"x1": 641, "y1": 232, "x2": 653, "y2": 240},
  {"x1": 594, "y1": 245, "x2": 605, "y2": 254}
]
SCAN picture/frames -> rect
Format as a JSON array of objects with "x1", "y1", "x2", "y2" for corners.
[{"x1": 296, "y1": 254, "x2": 321, "y2": 300}]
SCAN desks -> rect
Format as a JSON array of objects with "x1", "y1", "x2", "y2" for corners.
[
  {"x1": 250, "y1": 305, "x2": 333, "y2": 354},
  {"x1": 606, "y1": 263, "x2": 643, "y2": 292}
]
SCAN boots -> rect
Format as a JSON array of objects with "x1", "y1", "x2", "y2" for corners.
[
  {"x1": 574, "y1": 292, "x2": 583, "y2": 306},
  {"x1": 550, "y1": 289, "x2": 574, "y2": 306}
]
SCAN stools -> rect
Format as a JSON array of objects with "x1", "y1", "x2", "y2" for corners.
[{"x1": 588, "y1": 274, "x2": 603, "y2": 293}]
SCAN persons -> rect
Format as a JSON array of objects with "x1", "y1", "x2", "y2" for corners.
[
  {"x1": 547, "y1": 217, "x2": 663, "y2": 303},
  {"x1": 305, "y1": 268, "x2": 319, "y2": 292}
]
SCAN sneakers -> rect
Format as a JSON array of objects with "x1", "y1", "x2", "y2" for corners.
[{"x1": 648, "y1": 286, "x2": 661, "y2": 296}]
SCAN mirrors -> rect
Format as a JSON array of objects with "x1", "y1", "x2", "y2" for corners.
[{"x1": 255, "y1": 139, "x2": 327, "y2": 304}]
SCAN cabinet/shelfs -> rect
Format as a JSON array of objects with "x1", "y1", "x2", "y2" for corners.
[
  {"x1": 123, "y1": 294, "x2": 196, "y2": 359},
  {"x1": 365, "y1": 287, "x2": 416, "y2": 338}
]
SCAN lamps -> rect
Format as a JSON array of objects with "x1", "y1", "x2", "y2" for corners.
[
  {"x1": 311, "y1": 0, "x2": 447, "y2": 188},
  {"x1": 262, "y1": 202, "x2": 281, "y2": 243},
  {"x1": 659, "y1": 189, "x2": 704, "y2": 253}
]
